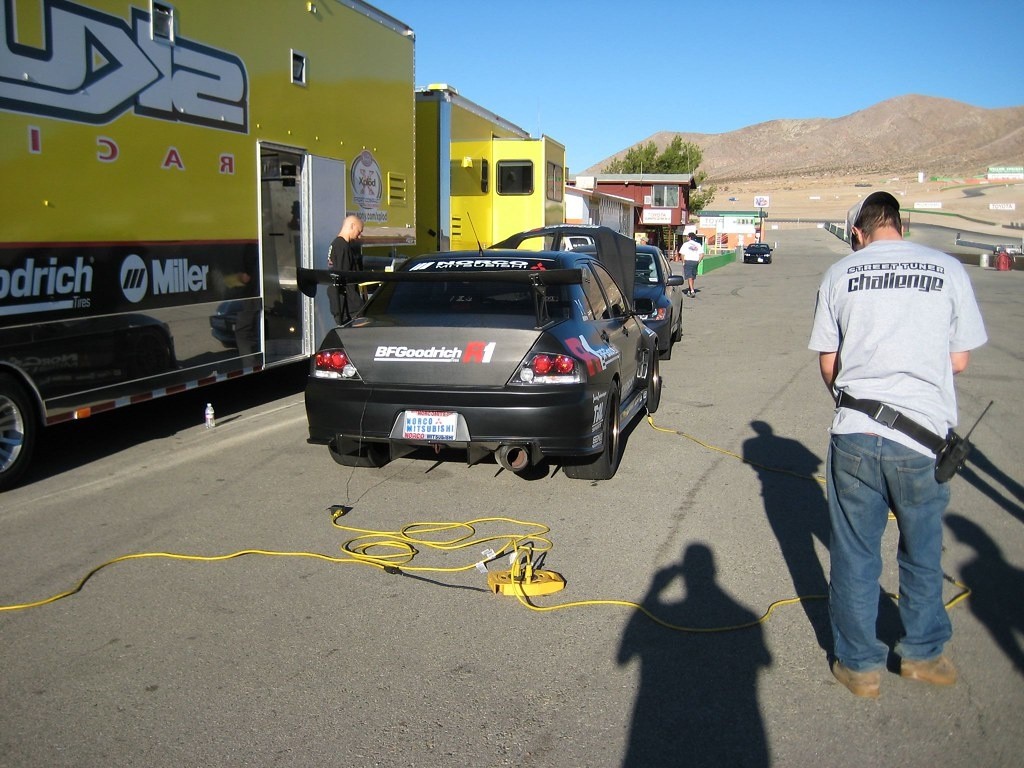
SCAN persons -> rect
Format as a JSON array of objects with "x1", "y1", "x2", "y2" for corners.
[
  {"x1": 235, "y1": 243, "x2": 260, "y2": 368},
  {"x1": 327, "y1": 215, "x2": 364, "y2": 326},
  {"x1": 809, "y1": 192, "x2": 989, "y2": 696},
  {"x1": 679, "y1": 233, "x2": 704, "y2": 297}
]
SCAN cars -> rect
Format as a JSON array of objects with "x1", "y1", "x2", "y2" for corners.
[
  {"x1": 294, "y1": 248, "x2": 662, "y2": 479},
  {"x1": 742, "y1": 243, "x2": 774, "y2": 264},
  {"x1": 566, "y1": 244, "x2": 685, "y2": 361}
]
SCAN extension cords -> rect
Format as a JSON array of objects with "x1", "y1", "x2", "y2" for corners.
[{"x1": 487, "y1": 569, "x2": 566, "y2": 597}]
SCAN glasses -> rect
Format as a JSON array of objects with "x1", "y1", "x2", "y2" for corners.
[{"x1": 687, "y1": 236, "x2": 690, "y2": 238}]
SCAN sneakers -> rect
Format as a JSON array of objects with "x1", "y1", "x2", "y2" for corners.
[
  {"x1": 832, "y1": 660, "x2": 882, "y2": 698},
  {"x1": 901, "y1": 650, "x2": 956, "y2": 685}
]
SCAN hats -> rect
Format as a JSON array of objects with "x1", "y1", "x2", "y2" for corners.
[
  {"x1": 640, "y1": 237, "x2": 649, "y2": 242},
  {"x1": 846, "y1": 190, "x2": 900, "y2": 251}
]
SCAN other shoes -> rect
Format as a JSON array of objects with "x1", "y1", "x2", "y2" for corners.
[
  {"x1": 690, "y1": 292, "x2": 695, "y2": 298},
  {"x1": 686, "y1": 292, "x2": 691, "y2": 296}
]
